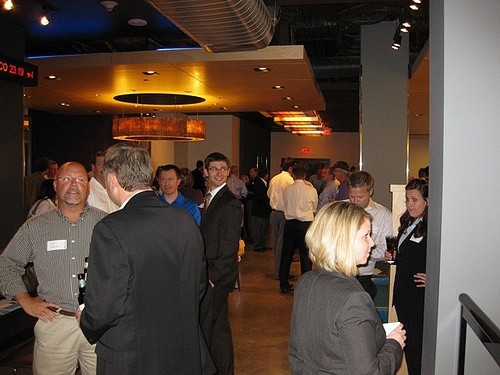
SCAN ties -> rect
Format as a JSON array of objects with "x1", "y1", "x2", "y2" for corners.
[{"x1": 204, "y1": 193, "x2": 212, "y2": 215}]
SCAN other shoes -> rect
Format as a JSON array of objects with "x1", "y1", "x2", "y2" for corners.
[
  {"x1": 254, "y1": 247, "x2": 273, "y2": 252},
  {"x1": 280, "y1": 283, "x2": 294, "y2": 294},
  {"x1": 292, "y1": 258, "x2": 299, "y2": 262},
  {"x1": 287, "y1": 274, "x2": 295, "y2": 279}
]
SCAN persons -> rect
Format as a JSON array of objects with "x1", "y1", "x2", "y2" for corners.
[
  {"x1": 288, "y1": 200, "x2": 406, "y2": 375},
  {"x1": 384, "y1": 178, "x2": 429, "y2": 375},
  {"x1": 0, "y1": 162, "x2": 110, "y2": 375},
  {"x1": 199, "y1": 152, "x2": 242, "y2": 375},
  {"x1": 342, "y1": 171, "x2": 393, "y2": 300},
  {"x1": 418, "y1": 166, "x2": 429, "y2": 182},
  {"x1": 25, "y1": 150, "x2": 357, "y2": 293},
  {"x1": 75, "y1": 142, "x2": 208, "y2": 375}
]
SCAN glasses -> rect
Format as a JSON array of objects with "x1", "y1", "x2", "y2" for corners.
[{"x1": 208, "y1": 166, "x2": 229, "y2": 174}]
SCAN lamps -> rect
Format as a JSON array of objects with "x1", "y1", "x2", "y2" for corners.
[
  {"x1": 260, "y1": 111, "x2": 330, "y2": 137},
  {"x1": 391, "y1": 0, "x2": 421, "y2": 51},
  {"x1": 113, "y1": 96, "x2": 207, "y2": 143}
]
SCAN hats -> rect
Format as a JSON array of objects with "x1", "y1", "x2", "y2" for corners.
[{"x1": 330, "y1": 161, "x2": 350, "y2": 171}]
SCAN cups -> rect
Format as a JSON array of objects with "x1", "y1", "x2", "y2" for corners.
[{"x1": 327, "y1": 195, "x2": 334, "y2": 202}]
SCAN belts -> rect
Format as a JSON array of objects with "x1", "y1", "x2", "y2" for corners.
[{"x1": 42, "y1": 299, "x2": 77, "y2": 316}]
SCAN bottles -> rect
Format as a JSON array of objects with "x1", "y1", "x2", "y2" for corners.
[
  {"x1": 77, "y1": 274, "x2": 87, "y2": 312},
  {"x1": 84, "y1": 257, "x2": 88, "y2": 281},
  {"x1": 385, "y1": 237, "x2": 394, "y2": 264}
]
